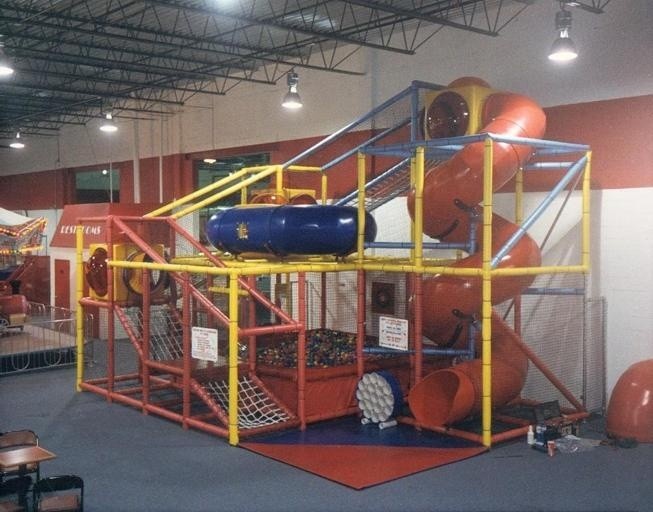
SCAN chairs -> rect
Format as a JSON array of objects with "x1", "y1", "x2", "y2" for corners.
[{"x1": 0, "y1": 430, "x2": 83, "y2": 512}]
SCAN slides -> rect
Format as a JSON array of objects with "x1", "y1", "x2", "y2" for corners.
[{"x1": 407, "y1": 92, "x2": 546, "y2": 427}]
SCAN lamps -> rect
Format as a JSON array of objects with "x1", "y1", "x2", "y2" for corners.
[
  {"x1": 282, "y1": 68, "x2": 302, "y2": 108},
  {"x1": 99, "y1": 103, "x2": 117, "y2": 132},
  {"x1": 548, "y1": 12, "x2": 577, "y2": 61},
  {"x1": 9, "y1": 126, "x2": 24, "y2": 148}
]
